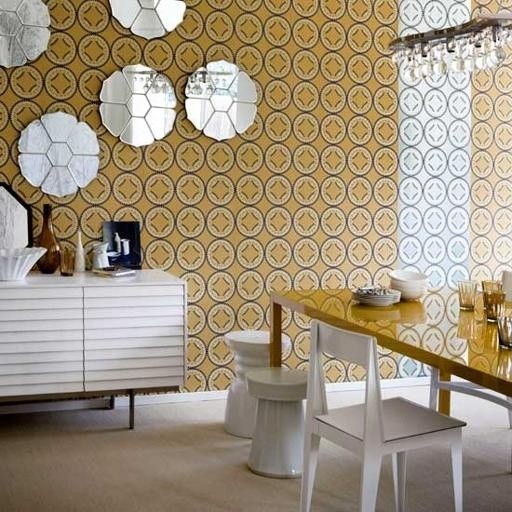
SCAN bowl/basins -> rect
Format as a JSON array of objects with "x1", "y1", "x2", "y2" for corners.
[
  {"x1": 388, "y1": 269, "x2": 429, "y2": 300},
  {"x1": 107, "y1": 251, "x2": 120, "y2": 260},
  {"x1": 0, "y1": 247, "x2": 48, "y2": 281}
]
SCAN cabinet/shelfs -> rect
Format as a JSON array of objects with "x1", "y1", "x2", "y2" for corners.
[{"x1": 1, "y1": 269, "x2": 187, "y2": 431}]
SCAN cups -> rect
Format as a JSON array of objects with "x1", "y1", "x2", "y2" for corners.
[
  {"x1": 481, "y1": 280, "x2": 506, "y2": 323},
  {"x1": 457, "y1": 280, "x2": 477, "y2": 311},
  {"x1": 497, "y1": 316, "x2": 512, "y2": 349},
  {"x1": 59, "y1": 248, "x2": 77, "y2": 277}
]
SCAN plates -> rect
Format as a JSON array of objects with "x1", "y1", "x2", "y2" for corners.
[{"x1": 351, "y1": 287, "x2": 402, "y2": 307}]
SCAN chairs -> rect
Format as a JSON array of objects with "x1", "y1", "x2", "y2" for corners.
[{"x1": 300, "y1": 319, "x2": 467, "y2": 511}]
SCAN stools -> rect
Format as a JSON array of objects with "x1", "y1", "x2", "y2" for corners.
[{"x1": 223, "y1": 329, "x2": 307, "y2": 479}]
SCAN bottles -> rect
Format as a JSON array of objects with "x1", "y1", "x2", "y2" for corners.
[
  {"x1": 74, "y1": 231, "x2": 86, "y2": 272},
  {"x1": 35, "y1": 203, "x2": 62, "y2": 274}
]
